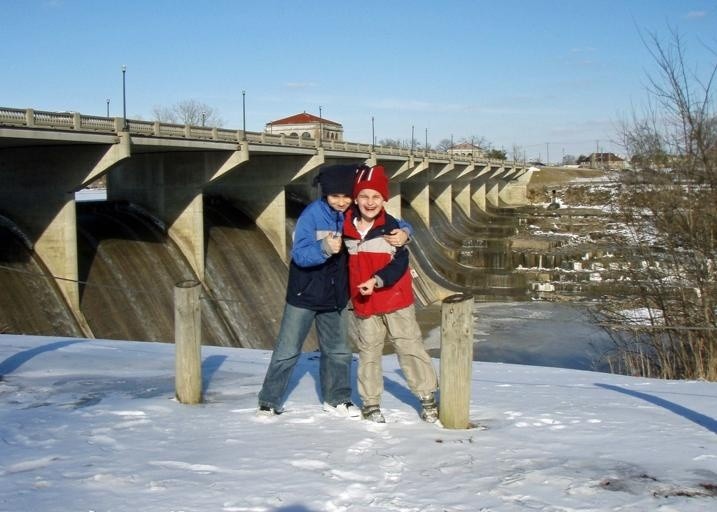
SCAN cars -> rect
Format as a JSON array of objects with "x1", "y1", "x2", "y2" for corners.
[{"x1": 527, "y1": 162, "x2": 545, "y2": 168}]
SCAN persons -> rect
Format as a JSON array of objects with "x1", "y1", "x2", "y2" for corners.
[
  {"x1": 342, "y1": 162, "x2": 440, "y2": 424},
  {"x1": 257, "y1": 164, "x2": 414, "y2": 420}
]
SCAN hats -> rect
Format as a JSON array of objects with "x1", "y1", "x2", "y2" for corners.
[
  {"x1": 312, "y1": 164, "x2": 356, "y2": 198},
  {"x1": 352, "y1": 165, "x2": 390, "y2": 201}
]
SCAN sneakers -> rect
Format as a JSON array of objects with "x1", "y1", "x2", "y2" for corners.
[
  {"x1": 419, "y1": 393, "x2": 438, "y2": 423},
  {"x1": 257, "y1": 407, "x2": 278, "y2": 419},
  {"x1": 360, "y1": 404, "x2": 385, "y2": 423},
  {"x1": 323, "y1": 401, "x2": 359, "y2": 418}
]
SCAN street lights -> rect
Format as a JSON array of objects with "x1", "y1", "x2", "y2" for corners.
[
  {"x1": 411, "y1": 125, "x2": 527, "y2": 165},
  {"x1": 201, "y1": 110, "x2": 205, "y2": 127},
  {"x1": 270, "y1": 120, "x2": 273, "y2": 134},
  {"x1": 318, "y1": 105, "x2": 322, "y2": 147},
  {"x1": 105, "y1": 97, "x2": 110, "y2": 118},
  {"x1": 121, "y1": 64, "x2": 128, "y2": 132},
  {"x1": 241, "y1": 87, "x2": 247, "y2": 141},
  {"x1": 371, "y1": 115, "x2": 374, "y2": 152}
]
